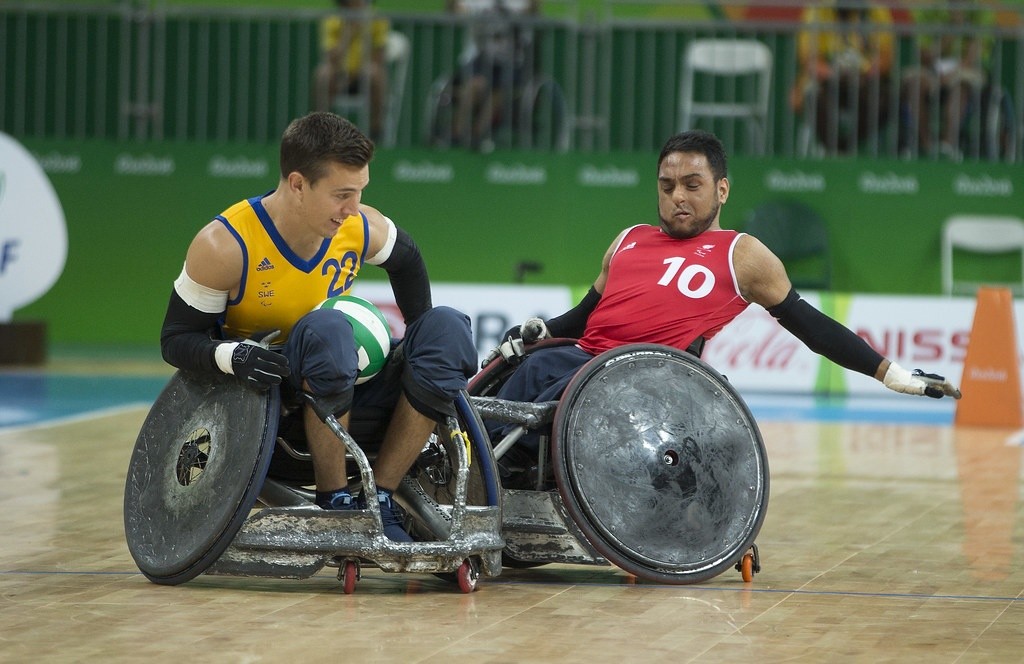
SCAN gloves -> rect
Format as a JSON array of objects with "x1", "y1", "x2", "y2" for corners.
[
  {"x1": 216, "y1": 327, "x2": 291, "y2": 392},
  {"x1": 882, "y1": 361, "x2": 962, "y2": 400},
  {"x1": 481, "y1": 316, "x2": 547, "y2": 366}
]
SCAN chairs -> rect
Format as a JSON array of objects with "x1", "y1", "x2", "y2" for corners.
[
  {"x1": 677, "y1": 39, "x2": 774, "y2": 155},
  {"x1": 332, "y1": 31, "x2": 409, "y2": 146},
  {"x1": 746, "y1": 197, "x2": 830, "y2": 290},
  {"x1": 940, "y1": 214, "x2": 1024, "y2": 299}
]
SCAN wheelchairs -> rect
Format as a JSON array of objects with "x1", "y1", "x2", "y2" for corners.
[
  {"x1": 121, "y1": 337, "x2": 503, "y2": 595},
  {"x1": 416, "y1": 71, "x2": 1022, "y2": 165},
  {"x1": 469, "y1": 337, "x2": 773, "y2": 585}
]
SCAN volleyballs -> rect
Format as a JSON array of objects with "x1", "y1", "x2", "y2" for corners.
[{"x1": 309, "y1": 295, "x2": 391, "y2": 386}]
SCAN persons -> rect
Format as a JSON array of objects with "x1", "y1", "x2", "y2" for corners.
[
  {"x1": 443, "y1": 0, "x2": 538, "y2": 143},
  {"x1": 904, "y1": 0, "x2": 997, "y2": 161},
  {"x1": 475, "y1": 129, "x2": 962, "y2": 486},
  {"x1": 795, "y1": 0, "x2": 898, "y2": 155},
  {"x1": 312, "y1": 0, "x2": 398, "y2": 144},
  {"x1": 157, "y1": 112, "x2": 479, "y2": 542}
]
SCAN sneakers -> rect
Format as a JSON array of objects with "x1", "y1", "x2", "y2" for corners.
[
  {"x1": 355, "y1": 495, "x2": 414, "y2": 543},
  {"x1": 324, "y1": 496, "x2": 358, "y2": 510}
]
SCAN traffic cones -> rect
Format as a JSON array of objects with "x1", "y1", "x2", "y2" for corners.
[{"x1": 956, "y1": 287, "x2": 1024, "y2": 432}]
